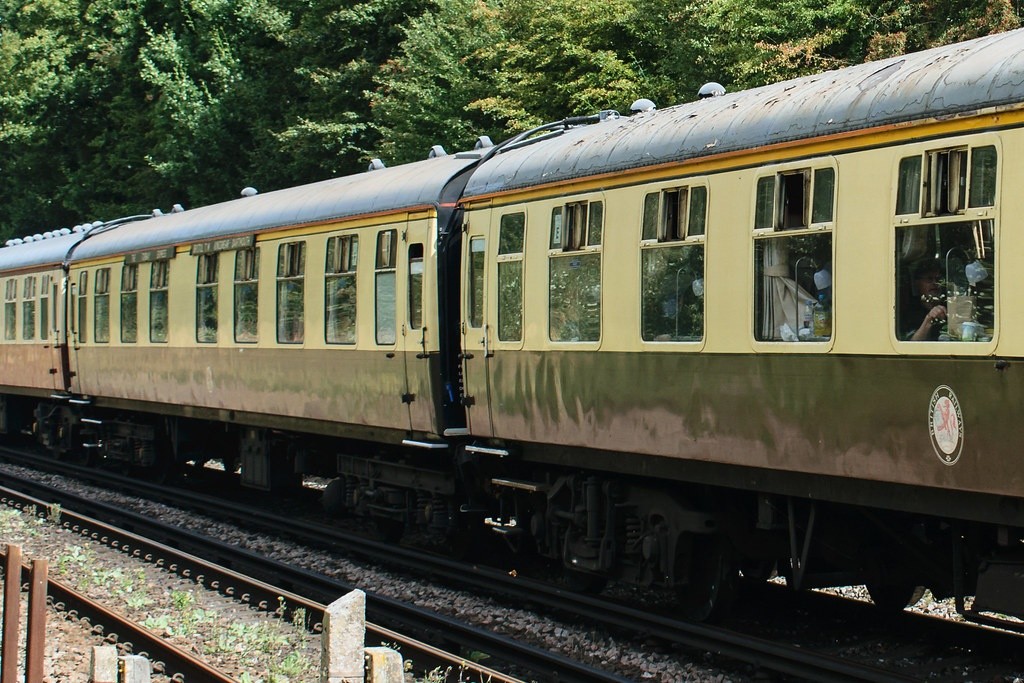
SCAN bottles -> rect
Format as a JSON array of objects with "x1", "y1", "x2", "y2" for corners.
[
  {"x1": 804, "y1": 301, "x2": 812, "y2": 328},
  {"x1": 814, "y1": 295, "x2": 831, "y2": 338}
]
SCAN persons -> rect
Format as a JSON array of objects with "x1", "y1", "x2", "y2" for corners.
[
  {"x1": 651, "y1": 294, "x2": 691, "y2": 341},
  {"x1": 900, "y1": 258, "x2": 946, "y2": 341}
]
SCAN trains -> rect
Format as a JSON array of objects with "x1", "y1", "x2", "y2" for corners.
[{"x1": 0, "y1": 29, "x2": 1024, "y2": 633}]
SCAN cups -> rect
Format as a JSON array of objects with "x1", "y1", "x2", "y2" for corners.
[{"x1": 962, "y1": 322, "x2": 975, "y2": 341}]
